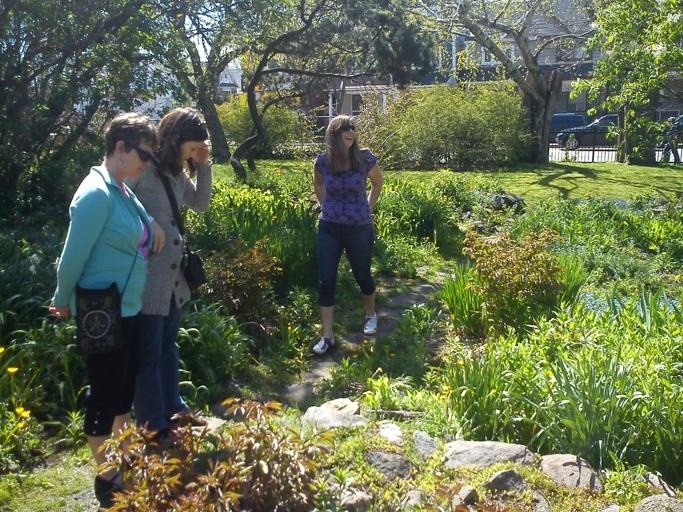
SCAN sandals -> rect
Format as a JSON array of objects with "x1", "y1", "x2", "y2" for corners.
[{"x1": 95, "y1": 470, "x2": 123, "y2": 488}]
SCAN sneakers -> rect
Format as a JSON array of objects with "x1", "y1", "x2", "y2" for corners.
[
  {"x1": 364, "y1": 312, "x2": 378, "y2": 335},
  {"x1": 313, "y1": 336, "x2": 335, "y2": 355}
]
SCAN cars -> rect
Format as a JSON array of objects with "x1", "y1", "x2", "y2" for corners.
[{"x1": 549, "y1": 112, "x2": 683, "y2": 148}]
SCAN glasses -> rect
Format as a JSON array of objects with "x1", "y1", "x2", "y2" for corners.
[
  {"x1": 342, "y1": 125, "x2": 355, "y2": 131},
  {"x1": 127, "y1": 142, "x2": 151, "y2": 161}
]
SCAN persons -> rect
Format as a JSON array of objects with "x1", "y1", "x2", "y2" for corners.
[
  {"x1": 308, "y1": 113, "x2": 385, "y2": 355},
  {"x1": 660, "y1": 116, "x2": 681, "y2": 164},
  {"x1": 48, "y1": 110, "x2": 167, "y2": 508},
  {"x1": 124, "y1": 106, "x2": 214, "y2": 451}
]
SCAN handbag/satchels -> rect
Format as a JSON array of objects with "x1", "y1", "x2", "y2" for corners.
[
  {"x1": 186, "y1": 249, "x2": 205, "y2": 287},
  {"x1": 76, "y1": 283, "x2": 121, "y2": 356}
]
